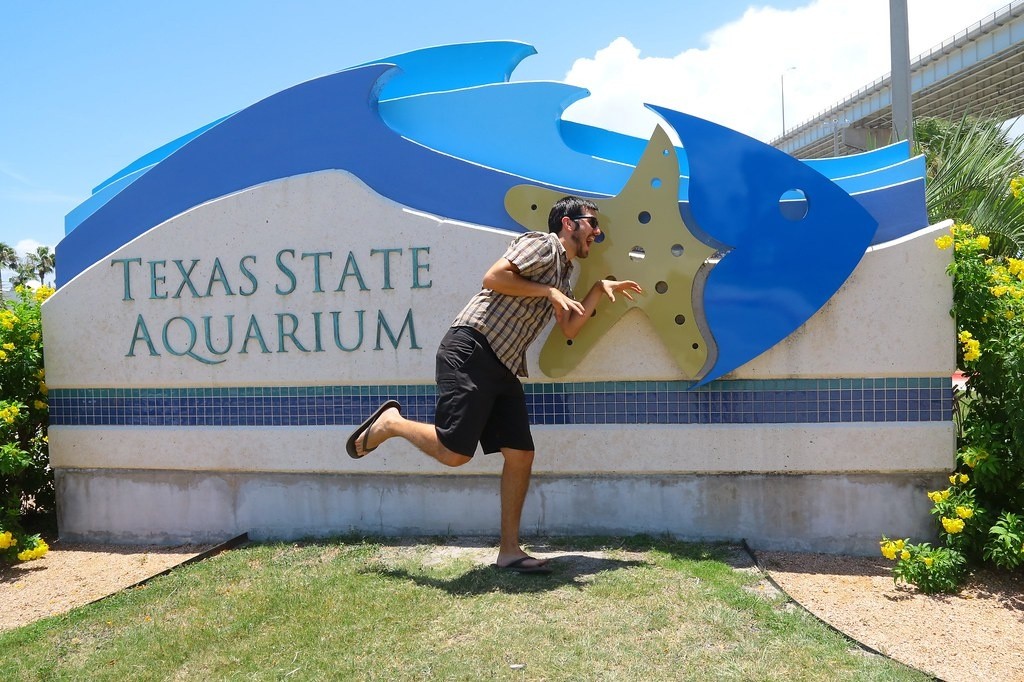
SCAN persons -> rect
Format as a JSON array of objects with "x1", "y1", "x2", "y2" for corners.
[{"x1": 346, "y1": 197, "x2": 643, "y2": 573}]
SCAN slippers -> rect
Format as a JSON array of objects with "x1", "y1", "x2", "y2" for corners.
[
  {"x1": 346, "y1": 399, "x2": 402, "y2": 460},
  {"x1": 490, "y1": 555, "x2": 553, "y2": 574}
]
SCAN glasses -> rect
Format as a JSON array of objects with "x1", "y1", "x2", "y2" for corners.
[{"x1": 570, "y1": 215, "x2": 599, "y2": 229}]
